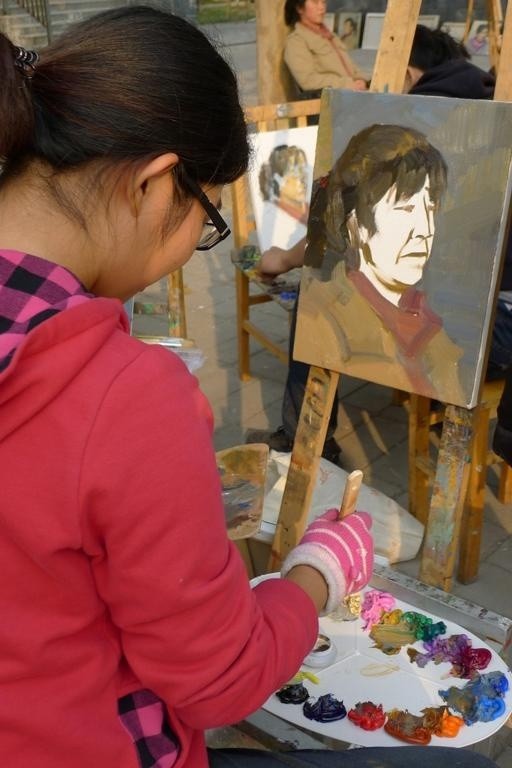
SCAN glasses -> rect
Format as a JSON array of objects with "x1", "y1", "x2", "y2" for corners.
[{"x1": 174, "y1": 162, "x2": 229, "y2": 251}]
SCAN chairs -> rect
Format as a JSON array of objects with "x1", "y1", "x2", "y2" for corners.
[{"x1": 231, "y1": 98, "x2": 320, "y2": 382}]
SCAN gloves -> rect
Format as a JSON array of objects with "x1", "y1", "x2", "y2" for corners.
[{"x1": 278, "y1": 509, "x2": 375, "y2": 614}]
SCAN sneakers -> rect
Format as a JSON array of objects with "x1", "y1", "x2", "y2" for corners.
[{"x1": 246, "y1": 426, "x2": 341, "y2": 464}]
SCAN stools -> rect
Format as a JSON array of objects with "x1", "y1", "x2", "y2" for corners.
[{"x1": 403, "y1": 380, "x2": 511, "y2": 586}]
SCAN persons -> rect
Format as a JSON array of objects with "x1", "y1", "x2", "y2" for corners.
[
  {"x1": 340, "y1": 18, "x2": 358, "y2": 49},
  {"x1": 259, "y1": 144, "x2": 311, "y2": 257},
  {"x1": 245, "y1": 24, "x2": 512, "y2": 472},
  {"x1": 284, "y1": 0, "x2": 374, "y2": 124},
  {"x1": 466, "y1": 23, "x2": 491, "y2": 56},
  {"x1": 293, "y1": 125, "x2": 465, "y2": 407},
  {"x1": 1, "y1": 4, "x2": 375, "y2": 767}
]
